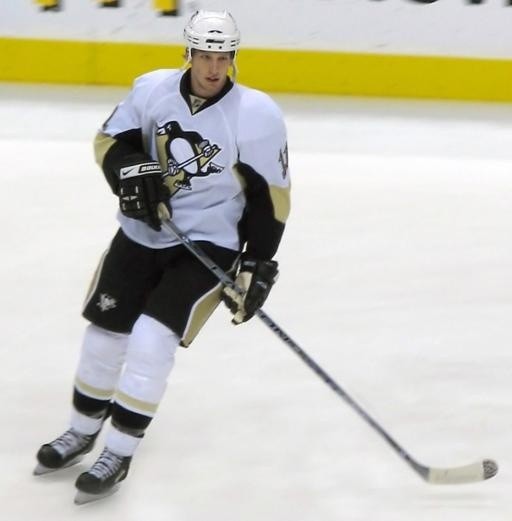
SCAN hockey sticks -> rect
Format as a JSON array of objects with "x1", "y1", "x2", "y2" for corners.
[{"x1": 158, "y1": 206, "x2": 497, "y2": 484}]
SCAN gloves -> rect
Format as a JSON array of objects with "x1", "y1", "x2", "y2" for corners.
[
  {"x1": 106, "y1": 145, "x2": 173, "y2": 232},
  {"x1": 220, "y1": 252, "x2": 279, "y2": 325}
]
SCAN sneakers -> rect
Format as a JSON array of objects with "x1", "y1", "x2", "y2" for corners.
[
  {"x1": 37, "y1": 426, "x2": 102, "y2": 469},
  {"x1": 75, "y1": 446, "x2": 132, "y2": 495}
]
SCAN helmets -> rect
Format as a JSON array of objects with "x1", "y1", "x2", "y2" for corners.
[{"x1": 183, "y1": 8, "x2": 241, "y2": 65}]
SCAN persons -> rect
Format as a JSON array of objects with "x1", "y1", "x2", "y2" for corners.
[{"x1": 37, "y1": 9, "x2": 291, "y2": 493}]
